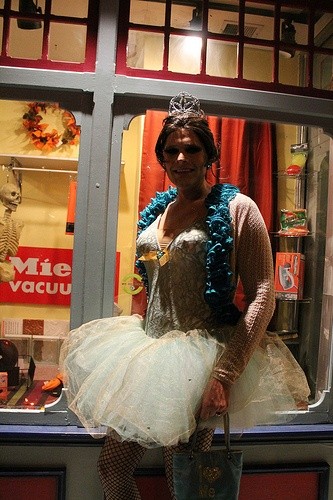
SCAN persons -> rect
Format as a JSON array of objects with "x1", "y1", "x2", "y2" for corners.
[{"x1": 59, "y1": 90, "x2": 313, "y2": 500}]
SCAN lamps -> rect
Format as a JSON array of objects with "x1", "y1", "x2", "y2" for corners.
[
  {"x1": 17, "y1": 0, "x2": 42, "y2": 30},
  {"x1": 277, "y1": 18, "x2": 299, "y2": 59}
]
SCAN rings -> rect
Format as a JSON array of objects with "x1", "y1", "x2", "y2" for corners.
[{"x1": 215, "y1": 412, "x2": 221, "y2": 416}]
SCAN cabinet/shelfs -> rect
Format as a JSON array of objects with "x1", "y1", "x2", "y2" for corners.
[{"x1": 273, "y1": 165, "x2": 310, "y2": 344}]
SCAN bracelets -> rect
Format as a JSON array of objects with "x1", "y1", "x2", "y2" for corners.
[{"x1": 212, "y1": 368, "x2": 235, "y2": 386}]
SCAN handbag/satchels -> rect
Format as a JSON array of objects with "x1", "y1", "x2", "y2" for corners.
[{"x1": 172, "y1": 413, "x2": 244, "y2": 499}]
